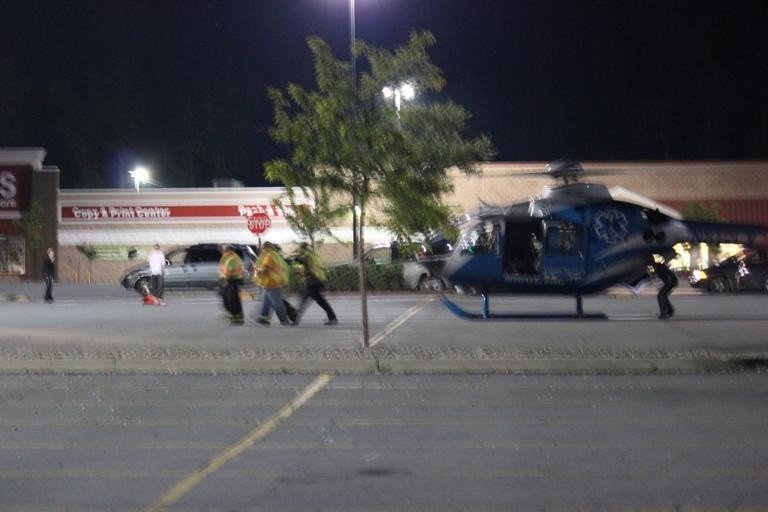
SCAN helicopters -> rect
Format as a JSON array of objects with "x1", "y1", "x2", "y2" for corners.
[{"x1": 420, "y1": 161, "x2": 768, "y2": 321}]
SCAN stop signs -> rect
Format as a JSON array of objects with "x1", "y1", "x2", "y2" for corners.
[{"x1": 248, "y1": 213, "x2": 271, "y2": 233}]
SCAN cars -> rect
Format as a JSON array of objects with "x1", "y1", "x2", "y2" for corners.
[
  {"x1": 687, "y1": 245, "x2": 768, "y2": 294},
  {"x1": 330, "y1": 242, "x2": 429, "y2": 291},
  {"x1": 120, "y1": 243, "x2": 258, "y2": 296}
]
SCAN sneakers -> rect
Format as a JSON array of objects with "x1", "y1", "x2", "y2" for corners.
[{"x1": 224, "y1": 312, "x2": 340, "y2": 328}]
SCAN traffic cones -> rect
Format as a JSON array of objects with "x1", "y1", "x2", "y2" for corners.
[{"x1": 144, "y1": 295, "x2": 167, "y2": 306}]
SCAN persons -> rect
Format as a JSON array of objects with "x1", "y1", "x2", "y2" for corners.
[
  {"x1": 146, "y1": 244, "x2": 165, "y2": 297},
  {"x1": 41, "y1": 247, "x2": 57, "y2": 301},
  {"x1": 390, "y1": 240, "x2": 401, "y2": 261},
  {"x1": 528, "y1": 231, "x2": 543, "y2": 256},
  {"x1": 215, "y1": 242, "x2": 339, "y2": 328},
  {"x1": 652, "y1": 263, "x2": 678, "y2": 318}
]
